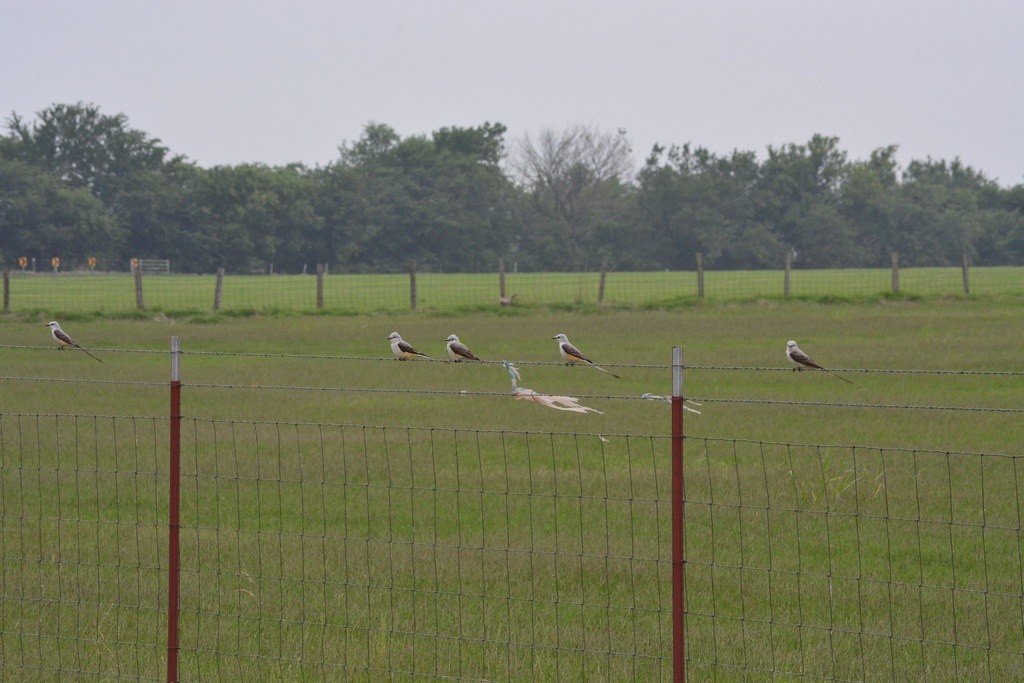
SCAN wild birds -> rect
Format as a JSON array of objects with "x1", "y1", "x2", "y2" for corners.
[
  {"x1": 45, "y1": 321, "x2": 104, "y2": 365},
  {"x1": 387, "y1": 333, "x2": 436, "y2": 363},
  {"x1": 552, "y1": 332, "x2": 620, "y2": 376},
  {"x1": 445, "y1": 333, "x2": 481, "y2": 363},
  {"x1": 786, "y1": 339, "x2": 852, "y2": 385}
]
